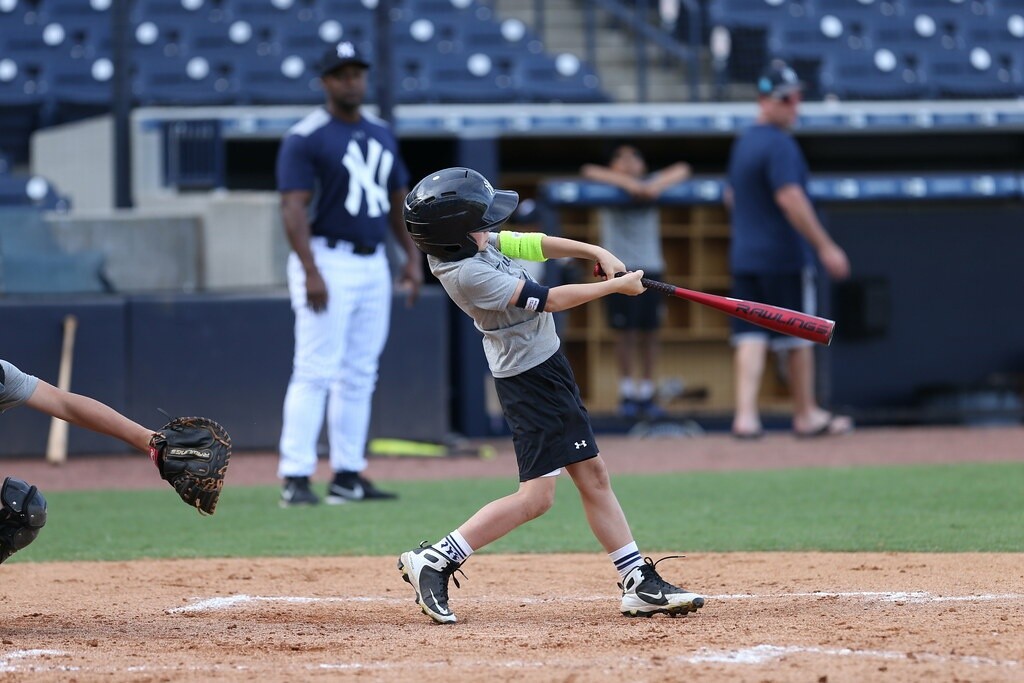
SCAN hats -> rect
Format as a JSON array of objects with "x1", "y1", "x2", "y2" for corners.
[
  {"x1": 317, "y1": 43, "x2": 372, "y2": 75},
  {"x1": 758, "y1": 62, "x2": 799, "y2": 96}
]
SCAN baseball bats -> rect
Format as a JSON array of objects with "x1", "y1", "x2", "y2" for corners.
[
  {"x1": 47, "y1": 315, "x2": 77, "y2": 467},
  {"x1": 592, "y1": 260, "x2": 837, "y2": 346},
  {"x1": 367, "y1": 436, "x2": 497, "y2": 460}
]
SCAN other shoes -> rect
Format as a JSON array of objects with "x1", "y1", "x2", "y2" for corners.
[
  {"x1": 622, "y1": 392, "x2": 668, "y2": 424},
  {"x1": 791, "y1": 413, "x2": 855, "y2": 437},
  {"x1": 737, "y1": 429, "x2": 767, "y2": 439}
]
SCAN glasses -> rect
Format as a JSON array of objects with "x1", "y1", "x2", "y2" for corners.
[{"x1": 780, "y1": 96, "x2": 791, "y2": 103}]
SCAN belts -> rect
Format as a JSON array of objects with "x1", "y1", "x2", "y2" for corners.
[{"x1": 327, "y1": 238, "x2": 376, "y2": 256}]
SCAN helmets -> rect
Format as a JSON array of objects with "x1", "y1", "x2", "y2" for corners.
[{"x1": 403, "y1": 166, "x2": 519, "y2": 262}]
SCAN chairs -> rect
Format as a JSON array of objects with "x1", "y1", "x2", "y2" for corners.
[
  {"x1": 0, "y1": 0, "x2": 610, "y2": 128},
  {"x1": 646, "y1": 0, "x2": 1024, "y2": 102}
]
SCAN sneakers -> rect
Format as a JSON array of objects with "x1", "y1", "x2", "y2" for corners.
[
  {"x1": 326, "y1": 480, "x2": 402, "y2": 504},
  {"x1": 616, "y1": 555, "x2": 705, "y2": 618},
  {"x1": 398, "y1": 540, "x2": 468, "y2": 624},
  {"x1": 278, "y1": 476, "x2": 324, "y2": 509}
]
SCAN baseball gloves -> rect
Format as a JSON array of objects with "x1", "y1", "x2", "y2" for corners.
[{"x1": 147, "y1": 415, "x2": 232, "y2": 516}]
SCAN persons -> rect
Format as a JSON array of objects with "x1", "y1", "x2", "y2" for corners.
[
  {"x1": 397, "y1": 167, "x2": 704, "y2": 625},
  {"x1": 721, "y1": 59, "x2": 853, "y2": 440},
  {"x1": 274, "y1": 37, "x2": 423, "y2": 507},
  {"x1": 581, "y1": 144, "x2": 690, "y2": 427},
  {"x1": 0, "y1": 358, "x2": 233, "y2": 563}
]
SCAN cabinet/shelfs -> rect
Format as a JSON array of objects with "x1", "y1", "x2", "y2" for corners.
[{"x1": 496, "y1": 171, "x2": 798, "y2": 420}]
